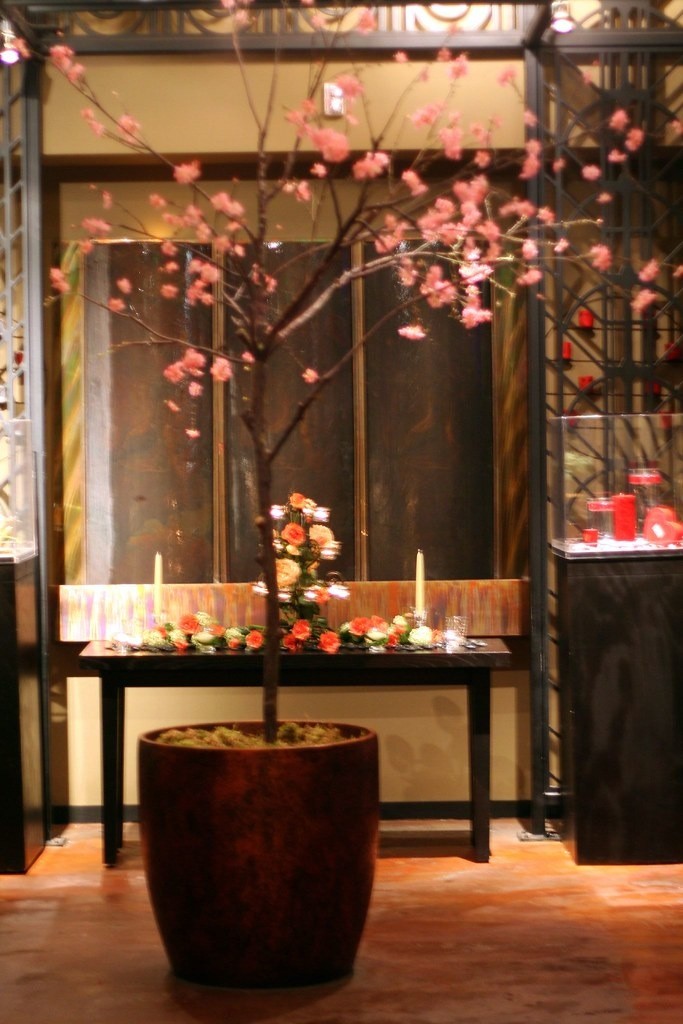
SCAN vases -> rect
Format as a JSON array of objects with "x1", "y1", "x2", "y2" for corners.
[{"x1": 137, "y1": 720, "x2": 379, "y2": 987}]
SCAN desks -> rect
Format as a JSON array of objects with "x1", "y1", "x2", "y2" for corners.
[{"x1": 52, "y1": 638, "x2": 510, "y2": 865}]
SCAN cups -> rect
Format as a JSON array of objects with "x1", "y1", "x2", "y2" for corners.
[
  {"x1": 112, "y1": 619, "x2": 136, "y2": 652},
  {"x1": 587, "y1": 497, "x2": 615, "y2": 541},
  {"x1": 627, "y1": 468, "x2": 663, "y2": 538},
  {"x1": 446, "y1": 616, "x2": 468, "y2": 645}
]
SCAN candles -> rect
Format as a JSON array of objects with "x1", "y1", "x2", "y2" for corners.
[
  {"x1": 415, "y1": 549, "x2": 427, "y2": 617},
  {"x1": 153, "y1": 551, "x2": 163, "y2": 619}
]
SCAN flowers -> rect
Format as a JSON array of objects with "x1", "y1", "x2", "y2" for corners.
[{"x1": 0, "y1": 0, "x2": 682, "y2": 743}]
